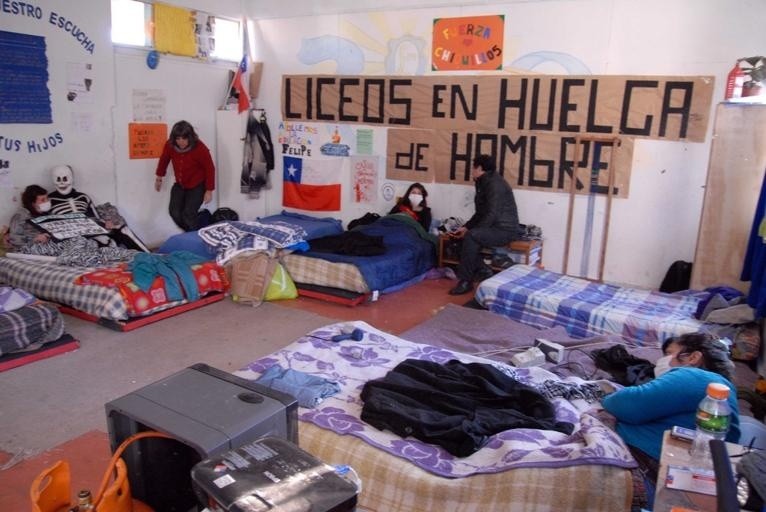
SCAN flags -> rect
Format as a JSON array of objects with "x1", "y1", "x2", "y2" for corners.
[
  {"x1": 282, "y1": 155, "x2": 344, "y2": 213},
  {"x1": 231, "y1": 55, "x2": 251, "y2": 115}
]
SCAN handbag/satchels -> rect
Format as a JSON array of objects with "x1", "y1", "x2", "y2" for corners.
[{"x1": 230, "y1": 251, "x2": 277, "y2": 309}]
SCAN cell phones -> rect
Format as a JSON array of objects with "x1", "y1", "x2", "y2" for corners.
[{"x1": 671, "y1": 425, "x2": 696, "y2": 443}]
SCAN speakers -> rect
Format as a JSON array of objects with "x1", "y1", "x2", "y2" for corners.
[
  {"x1": 534, "y1": 337, "x2": 564, "y2": 364},
  {"x1": 510, "y1": 347, "x2": 546, "y2": 369}
]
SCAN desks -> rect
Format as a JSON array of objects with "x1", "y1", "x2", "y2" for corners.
[{"x1": 438, "y1": 229, "x2": 546, "y2": 283}]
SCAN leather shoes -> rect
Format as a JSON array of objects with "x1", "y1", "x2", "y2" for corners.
[{"x1": 448, "y1": 280, "x2": 474, "y2": 296}]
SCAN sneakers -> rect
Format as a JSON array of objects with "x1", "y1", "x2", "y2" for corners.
[
  {"x1": 478, "y1": 269, "x2": 494, "y2": 282},
  {"x1": 203, "y1": 208, "x2": 213, "y2": 224}
]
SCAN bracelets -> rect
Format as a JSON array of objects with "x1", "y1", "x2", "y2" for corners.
[{"x1": 156, "y1": 178, "x2": 162, "y2": 183}]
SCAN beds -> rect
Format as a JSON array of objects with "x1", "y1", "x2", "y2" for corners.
[
  {"x1": 229, "y1": 264, "x2": 766, "y2": 512},
  {"x1": 163, "y1": 210, "x2": 446, "y2": 310},
  {"x1": 1, "y1": 229, "x2": 230, "y2": 372}
]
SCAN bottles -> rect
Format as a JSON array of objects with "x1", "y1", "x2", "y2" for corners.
[{"x1": 689, "y1": 383, "x2": 731, "y2": 472}]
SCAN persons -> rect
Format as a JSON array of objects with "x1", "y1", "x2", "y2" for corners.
[
  {"x1": 389, "y1": 182, "x2": 432, "y2": 234},
  {"x1": 3, "y1": 163, "x2": 152, "y2": 254},
  {"x1": 154, "y1": 120, "x2": 215, "y2": 232},
  {"x1": 448, "y1": 154, "x2": 521, "y2": 296},
  {"x1": 595, "y1": 331, "x2": 742, "y2": 511},
  {"x1": 7, "y1": 184, "x2": 108, "y2": 266}
]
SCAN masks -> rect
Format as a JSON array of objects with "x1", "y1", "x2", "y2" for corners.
[
  {"x1": 408, "y1": 194, "x2": 423, "y2": 204},
  {"x1": 34, "y1": 201, "x2": 51, "y2": 213},
  {"x1": 653, "y1": 352, "x2": 693, "y2": 379}
]
